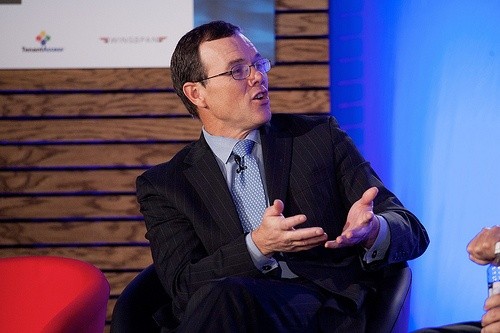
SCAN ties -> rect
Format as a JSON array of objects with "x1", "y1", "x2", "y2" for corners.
[{"x1": 231, "y1": 139, "x2": 267, "y2": 233}]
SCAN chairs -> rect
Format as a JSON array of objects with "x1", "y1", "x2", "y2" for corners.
[
  {"x1": 110, "y1": 261, "x2": 413, "y2": 332},
  {"x1": 0, "y1": 256, "x2": 111, "y2": 332}
]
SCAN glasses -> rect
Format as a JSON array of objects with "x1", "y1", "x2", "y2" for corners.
[{"x1": 200, "y1": 57, "x2": 271, "y2": 80}]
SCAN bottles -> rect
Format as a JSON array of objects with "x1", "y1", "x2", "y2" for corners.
[{"x1": 486, "y1": 241, "x2": 500, "y2": 297}]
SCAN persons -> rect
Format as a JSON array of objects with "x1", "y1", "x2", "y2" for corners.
[
  {"x1": 466, "y1": 226, "x2": 500, "y2": 333},
  {"x1": 135, "y1": 21, "x2": 430, "y2": 333}
]
